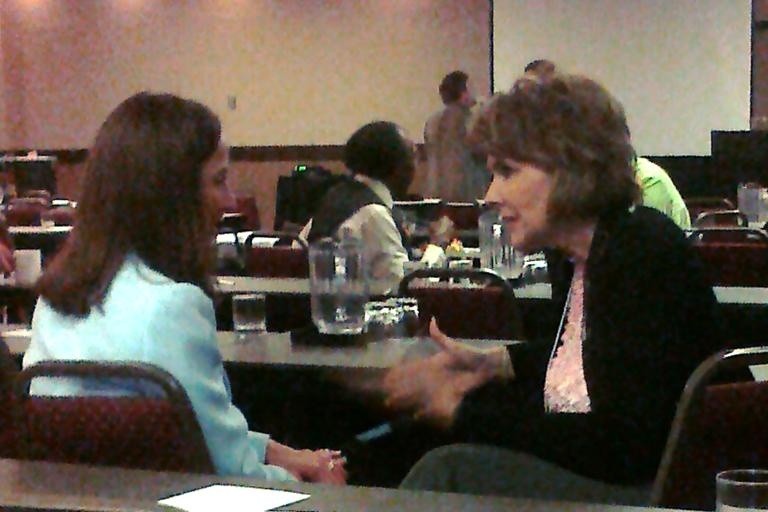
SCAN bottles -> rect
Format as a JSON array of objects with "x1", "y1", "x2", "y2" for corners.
[
  {"x1": 737, "y1": 183, "x2": 766, "y2": 229},
  {"x1": 308, "y1": 237, "x2": 366, "y2": 335},
  {"x1": 479, "y1": 211, "x2": 524, "y2": 279}
]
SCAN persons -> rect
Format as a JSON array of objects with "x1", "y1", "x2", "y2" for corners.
[
  {"x1": 630, "y1": 157, "x2": 692, "y2": 231},
  {"x1": 21, "y1": 93, "x2": 348, "y2": 486},
  {"x1": 524, "y1": 59, "x2": 556, "y2": 75},
  {"x1": 381, "y1": 75, "x2": 755, "y2": 485},
  {"x1": 423, "y1": 70, "x2": 488, "y2": 202},
  {"x1": 291, "y1": 117, "x2": 420, "y2": 281}
]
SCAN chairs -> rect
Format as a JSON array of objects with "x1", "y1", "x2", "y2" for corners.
[
  {"x1": 6, "y1": 359, "x2": 218, "y2": 474},
  {"x1": 646, "y1": 345, "x2": 768, "y2": 508},
  {"x1": 1, "y1": 187, "x2": 767, "y2": 340}
]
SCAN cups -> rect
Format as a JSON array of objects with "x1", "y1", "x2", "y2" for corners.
[
  {"x1": 714, "y1": 468, "x2": 768, "y2": 512},
  {"x1": 232, "y1": 294, "x2": 267, "y2": 338},
  {"x1": 12, "y1": 250, "x2": 42, "y2": 286}
]
